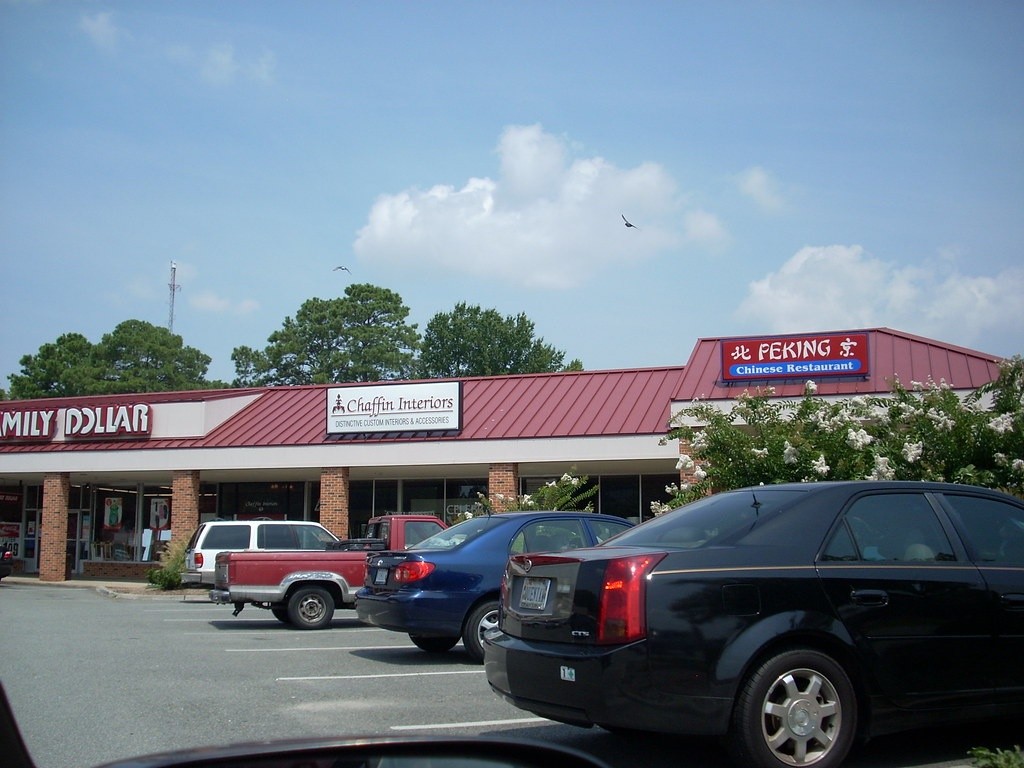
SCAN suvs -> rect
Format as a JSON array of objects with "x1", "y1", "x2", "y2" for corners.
[{"x1": 180, "y1": 520, "x2": 349, "y2": 591}]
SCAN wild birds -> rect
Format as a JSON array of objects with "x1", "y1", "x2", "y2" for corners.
[
  {"x1": 332, "y1": 266, "x2": 351, "y2": 274},
  {"x1": 622, "y1": 214, "x2": 639, "y2": 230}
]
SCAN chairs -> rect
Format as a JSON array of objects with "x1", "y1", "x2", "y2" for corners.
[
  {"x1": 533, "y1": 534, "x2": 560, "y2": 550},
  {"x1": 883, "y1": 504, "x2": 933, "y2": 561}
]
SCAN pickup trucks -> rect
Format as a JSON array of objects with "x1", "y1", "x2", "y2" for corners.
[{"x1": 208, "y1": 514, "x2": 456, "y2": 631}]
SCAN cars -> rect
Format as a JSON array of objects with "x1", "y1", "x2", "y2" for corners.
[
  {"x1": 484, "y1": 481, "x2": 1024, "y2": 768},
  {"x1": 0, "y1": 545, "x2": 13, "y2": 581},
  {"x1": 354, "y1": 510, "x2": 648, "y2": 666}
]
